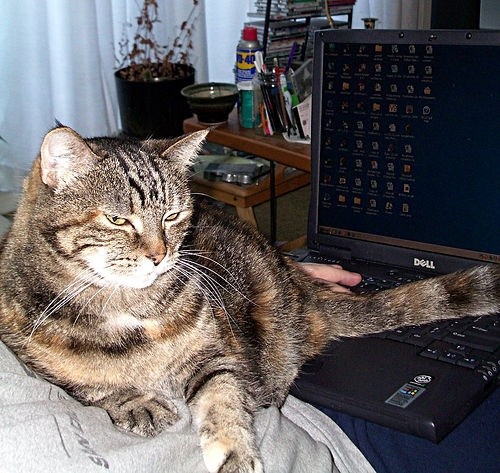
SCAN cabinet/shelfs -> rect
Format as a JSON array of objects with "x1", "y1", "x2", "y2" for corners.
[{"x1": 241, "y1": 0, "x2": 356, "y2": 72}]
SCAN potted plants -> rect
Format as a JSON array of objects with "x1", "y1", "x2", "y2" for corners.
[{"x1": 112, "y1": 0, "x2": 203, "y2": 140}]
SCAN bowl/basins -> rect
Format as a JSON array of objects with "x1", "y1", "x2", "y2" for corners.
[{"x1": 180, "y1": 82, "x2": 240, "y2": 126}]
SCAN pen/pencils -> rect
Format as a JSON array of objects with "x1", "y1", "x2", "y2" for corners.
[
  {"x1": 285, "y1": 42, "x2": 297, "y2": 72},
  {"x1": 252, "y1": 51, "x2": 303, "y2": 138}
]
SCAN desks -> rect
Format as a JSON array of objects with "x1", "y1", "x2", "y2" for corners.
[
  {"x1": 184, "y1": 107, "x2": 311, "y2": 179},
  {"x1": 183, "y1": 169, "x2": 310, "y2": 250}
]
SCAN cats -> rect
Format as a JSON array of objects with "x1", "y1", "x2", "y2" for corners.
[{"x1": 0, "y1": 125, "x2": 500, "y2": 473}]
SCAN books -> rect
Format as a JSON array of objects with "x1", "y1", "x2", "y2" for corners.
[{"x1": 240, "y1": 0, "x2": 357, "y2": 73}]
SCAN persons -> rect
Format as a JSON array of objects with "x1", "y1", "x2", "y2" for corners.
[{"x1": 0, "y1": 261, "x2": 499, "y2": 473}]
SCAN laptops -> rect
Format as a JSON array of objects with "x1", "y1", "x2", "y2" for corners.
[{"x1": 289, "y1": 29, "x2": 500, "y2": 443}]
